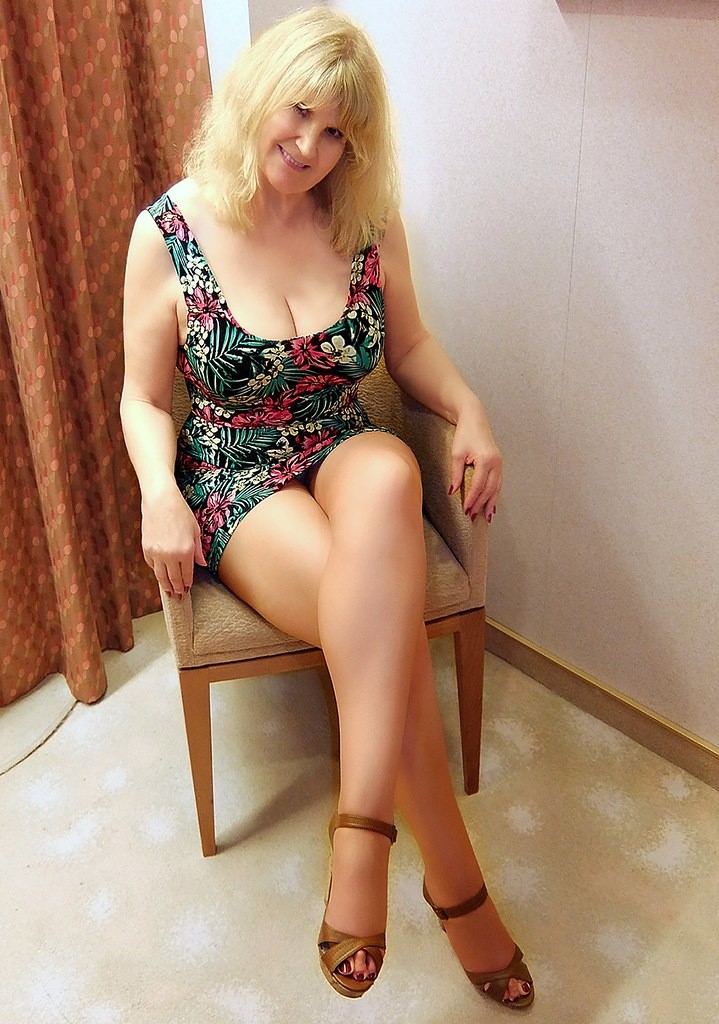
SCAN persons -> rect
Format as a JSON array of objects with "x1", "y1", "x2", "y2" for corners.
[{"x1": 120, "y1": 8, "x2": 539, "y2": 1009}]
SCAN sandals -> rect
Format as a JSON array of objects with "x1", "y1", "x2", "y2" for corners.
[
  {"x1": 421, "y1": 875, "x2": 534, "y2": 1010},
  {"x1": 317, "y1": 811, "x2": 398, "y2": 999}
]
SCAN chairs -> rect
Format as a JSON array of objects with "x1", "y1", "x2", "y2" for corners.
[{"x1": 159, "y1": 352, "x2": 491, "y2": 858}]
